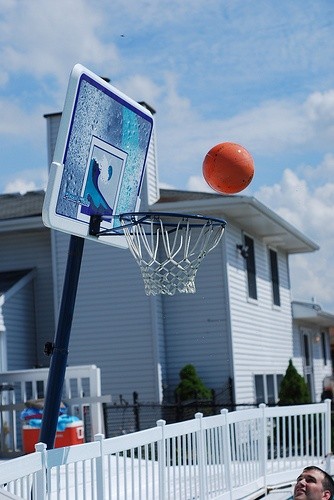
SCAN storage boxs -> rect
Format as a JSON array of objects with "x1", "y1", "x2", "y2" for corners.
[{"x1": 23, "y1": 419, "x2": 85, "y2": 454}]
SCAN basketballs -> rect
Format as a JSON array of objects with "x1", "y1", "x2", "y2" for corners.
[{"x1": 201, "y1": 141, "x2": 255, "y2": 196}]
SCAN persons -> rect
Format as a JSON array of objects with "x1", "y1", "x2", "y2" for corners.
[{"x1": 293, "y1": 466, "x2": 334, "y2": 500}]
